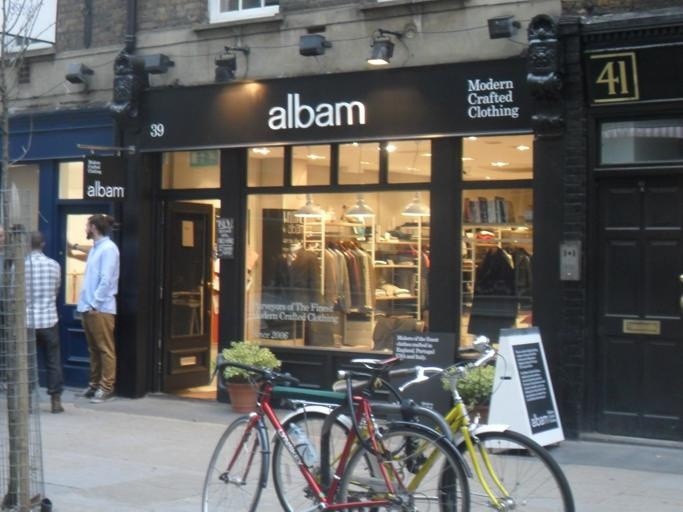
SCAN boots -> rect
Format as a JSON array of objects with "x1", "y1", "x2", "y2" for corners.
[{"x1": 50, "y1": 394, "x2": 64, "y2": 413}]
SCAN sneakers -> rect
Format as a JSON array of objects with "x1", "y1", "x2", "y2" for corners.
[
  {"x1": 90, "y1": 389, "x2": 116, "y2": 402},
  {"x1": 75, "y1": 386, "x2": 96, "y2": 398}
]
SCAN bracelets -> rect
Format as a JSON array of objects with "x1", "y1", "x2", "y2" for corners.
[{"x1": 72, "y1": 243, "x2": 78, "y2": 250}]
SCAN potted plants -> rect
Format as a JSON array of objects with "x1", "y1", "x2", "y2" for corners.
[
  {"x1": 218, "y1": 339, "x2": 283, "y2": 413},
  {"x1": 439, "y1": 361, "x2": 495, "y2": 426}
]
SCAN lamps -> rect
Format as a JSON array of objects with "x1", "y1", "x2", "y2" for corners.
[
  {"x1": 486, "y1": 13, "x2": 523, "y2": 42},
  {"x1": 400, "y1": 141, "x2": 431, "y2": 216},
  {"x1": 208, "y1": 43, "x2": 252, "y2": 84},
  {"x1": 296, "y1": 34, "x2": 334, "y2": 57},
  {"x1": 63, "y1": 62, "x2": 95, "y2": 86},
  {"x1": 364, "y1": 28, "x2": 409, "y2": 68},
  {"x1": 344, "y1": 142, "x2": 377, "y2": 218},
  {"x1": 141, "y1": 52, "x2": 173, "y2": 75},
  {"x1": 294, "y1": 144, "x2": 328, "y2": 220}
]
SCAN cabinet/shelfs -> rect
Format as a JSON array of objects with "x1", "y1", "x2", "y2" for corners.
[{"x1": 260, "y1": 206, "x2": 534, "y2": 351}]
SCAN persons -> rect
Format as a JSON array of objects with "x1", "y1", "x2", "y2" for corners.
[
  {"x1": 67, "y1": 240, "x2": 93, "y2": 262},
  {"x1": 8, "y1": 230, "x2": 65, "y2": 413},
  {"x1": 0, "y1": 221, "x2": 28, "y2": 392},
  {"x1": 76, "y1": 214, "x2": 120, "y2": 404}
]
